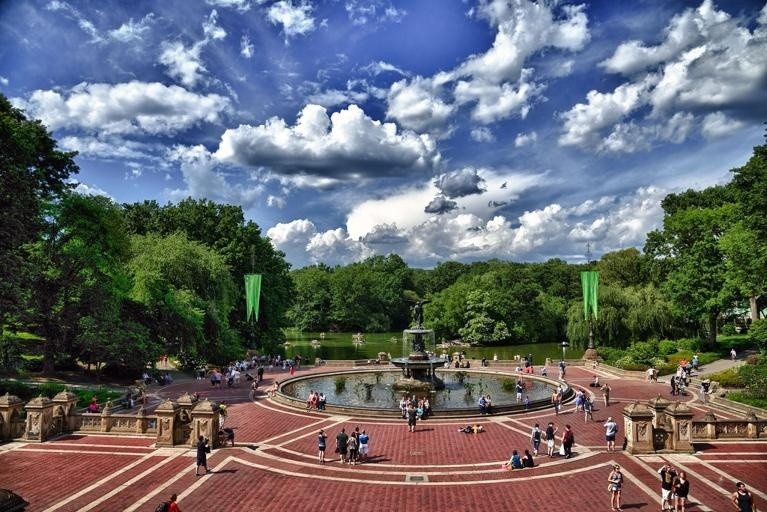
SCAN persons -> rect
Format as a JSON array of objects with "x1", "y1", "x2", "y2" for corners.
[
  {"x1": 607, "y1": 465, "x2": 624, "y2": 512},
  {"x1": 642, "y1": 347, "x2": 737, "y2": 396},
  {"x1": 731, "y1": 482, "x2": 757, "y2": 512},
  {"x1": 207, "y1": 353, "x2": 301, "y2": 397},
  {"x1": 89, "y1": 398, "x2": 101, "y2": 413},
  {"x1": 478, "y1": 395, "x2": 493, "y2": 415},
  {"x1": 445, "y1": 349, "x2": 486, "y2": 368},
  {"x1": 317, "y1": 425, "x2": 369, "y2": 467},
  {"x1": 127, "y1": 393, "x2": 134, "y2": 408},
  {"x1": 305, "y1": 390, "x2": 327, "y2": 413},
  {"x1": 160, "y1": 355, "x2": 163, "y2": 366},
  {"x1": 193, "y1": 401, "x2": 235, "y2": 478},
  {"x1": 409, "y1": 301, "x2": 432, "y2": 327},
  {"x1": 142, "y1": 371, "x2": 173, "y2": 386},
  {"x1": 163, "y1": 355, "x2": 168, "y2": 367},
  {"x1": 657, "y1": 464, "x2": 690, "y2": 511},
  {"x1": 399, "y1": 395, "x2": 432, "y2": 433},
  {"x1": 165, "y1": 493, "x2": 182, "y2": 512},
  {"x1": 506, "y1": 352, "x2": 618, "y2": 472}
]
